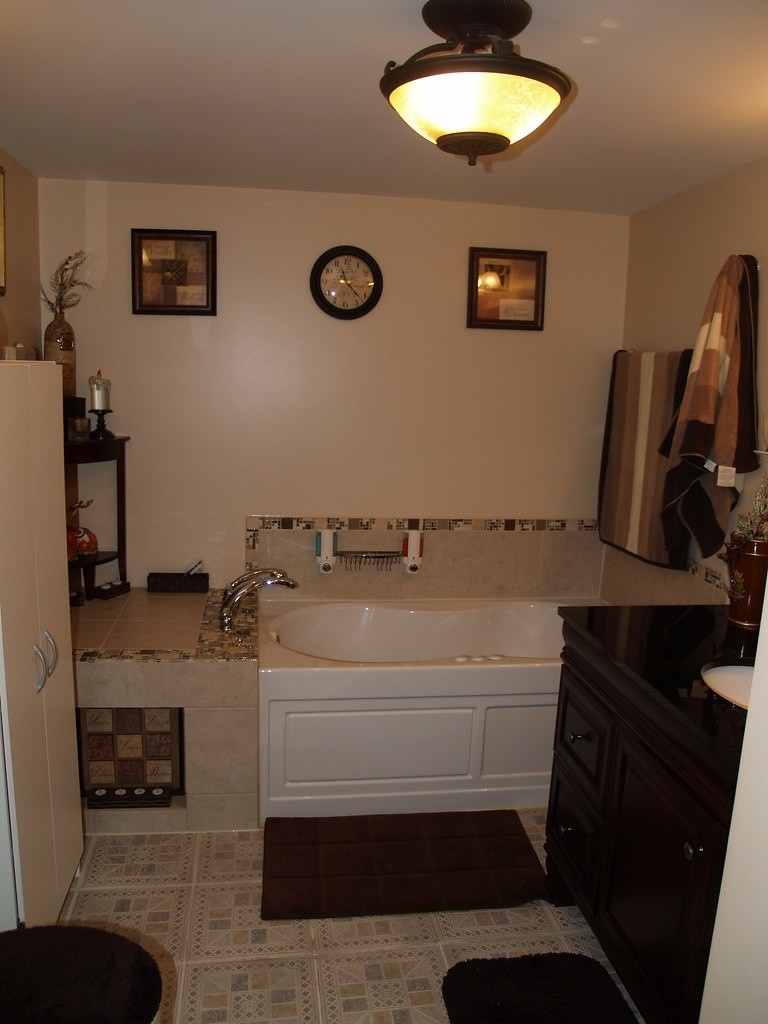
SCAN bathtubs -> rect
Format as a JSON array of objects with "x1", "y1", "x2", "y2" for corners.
[{"x1": 259, "y1": 600, "x2": 605, "y2": 667}]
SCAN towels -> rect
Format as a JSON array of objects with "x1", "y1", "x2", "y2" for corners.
[
  {"x1": 657, "y1": 253, "x2": 760, "y2": 571},
  {"x1": 598, "y1": 348, "x2": 702, "y2": 570}
]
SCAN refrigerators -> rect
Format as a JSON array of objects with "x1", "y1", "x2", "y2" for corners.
[{"x1": 1, "y1": 357, "x2": 84, "y2": 937}]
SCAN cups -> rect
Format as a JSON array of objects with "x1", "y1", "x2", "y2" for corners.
[{"x1": 69, "y1": 418, "x2": 91, "y2": 441}]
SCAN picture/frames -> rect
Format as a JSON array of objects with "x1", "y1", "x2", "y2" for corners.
[{"x1": 129, "y1": 228, "x2": 217, "y2": 316}]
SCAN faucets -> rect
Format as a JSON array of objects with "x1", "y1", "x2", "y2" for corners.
[{"x1": 219, "y1": 568, "x2": 300, "y2": 632}]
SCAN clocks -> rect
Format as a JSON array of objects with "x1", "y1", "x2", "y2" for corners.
[{"x1": 310, "y1": 245, "x2": 383, "y2": 320}]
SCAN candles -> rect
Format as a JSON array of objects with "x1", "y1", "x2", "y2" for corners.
[{"x1": 89, "y1": 370, "x2": 111, "y2": 410}]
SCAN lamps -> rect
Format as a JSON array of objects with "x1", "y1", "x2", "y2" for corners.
[{"x1": 377, "y1": 0, "x2": 572, "y2": 168}]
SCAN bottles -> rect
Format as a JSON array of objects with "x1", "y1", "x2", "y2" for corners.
[{"x1": 44, "y1": 312, "x2": 76, "y2": 399}]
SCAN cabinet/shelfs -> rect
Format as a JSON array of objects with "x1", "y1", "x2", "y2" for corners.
[
  {"x1": 61, "y1": 435, "x2": 131, "y2": 601},
  {"x1": 543, "y1": 620, "x2": 738, "y2": 1023},
  {"x1": 0, "y1": 359, "x2": 86, "y2": 930}
]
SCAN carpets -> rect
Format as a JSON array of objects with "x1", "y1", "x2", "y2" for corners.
[
  {"x1": 441, "y1": 951, "x2": 642, "y2": 1024},
  {"x1": 258, "y1": 809, "x2": 547, "y2": 921}
]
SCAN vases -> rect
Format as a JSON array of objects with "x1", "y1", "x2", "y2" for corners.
[
  {"x1": 44, "y1": 312, "x2": 77, "y2": 398},
  {"x1": 726, "y1": 531, "x2": 768, "y2": 629}
]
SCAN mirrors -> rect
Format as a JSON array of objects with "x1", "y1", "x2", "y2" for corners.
[{"x1": 468, "y1": 247, "x2": 548, "y2": 331}]
